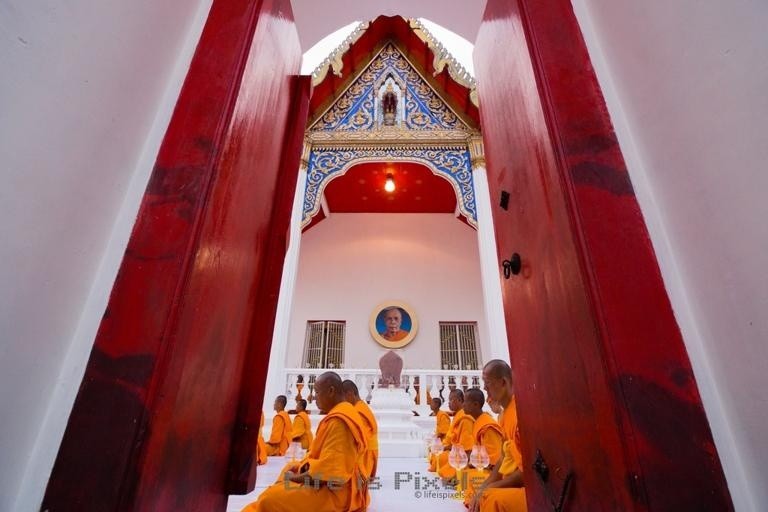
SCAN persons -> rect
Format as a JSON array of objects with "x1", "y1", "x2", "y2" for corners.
[
  {"x1": 265, "y1": 395, "x2": 292, "y2": 456},
  {"x1": 294, "y1": 375, "x2": 312, "y2": 402},
  {"x1": 406, "y1": 375, "x2": 434, "y2": 419},
  {"x1": 291, "y1": 397, "x2": 313, "y2": 451},
  {"x1": 341, "y1": 380, "x2": 378, "y2": 484},
  {"x1": 239, "y1": 371, "x2": 371, "y2": 511},
  {"x1": 255, "y1": 412, "x2": 266, "y2": 464},
  {"x1": 428, "y1": 360, "x2": 527, "y2": 511},
  {"x1": 379, "y1": 307, "x2": 407, "y2": 342}
]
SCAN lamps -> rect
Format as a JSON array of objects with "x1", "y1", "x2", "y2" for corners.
[{"x1": 381, "y1": 171, "x2": 399, "y2": 195}]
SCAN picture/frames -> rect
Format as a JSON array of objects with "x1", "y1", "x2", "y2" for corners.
[{"x1": 367, "y1": 299, "x2": 421, "y2": 350}]
